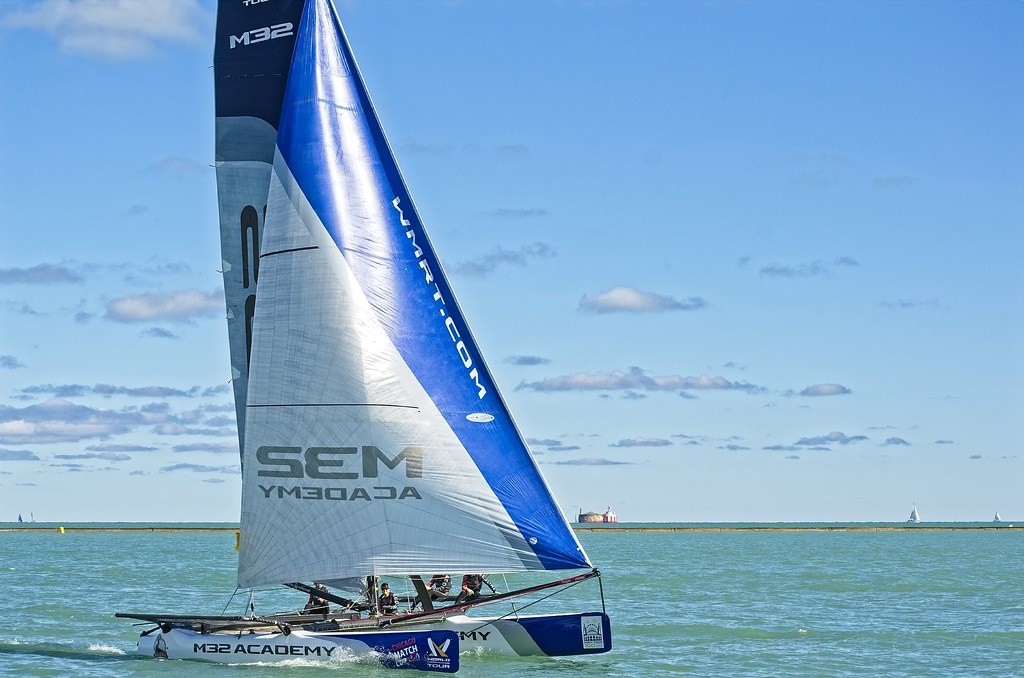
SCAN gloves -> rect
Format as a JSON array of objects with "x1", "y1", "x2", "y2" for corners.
[
  {"x1": 425, "y1": 585, "x2": 432, "y2": 591},
  {"x1": 495, "y1": 591, "x2": 501, "y2": 596},
  {"x1": 466, "y1": 589, "x2": 474, "y2": 597}
]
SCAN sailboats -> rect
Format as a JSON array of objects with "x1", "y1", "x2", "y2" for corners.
[{"x1": 109, "y1": 0, "x2": 615, "y2": 675}]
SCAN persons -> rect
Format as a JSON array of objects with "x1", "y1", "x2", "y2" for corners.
[
  {"x1": 299, "y1": 583, "x2": 331, "y2": 614},
  {"x1": 378, "y1": 583, "x2": 399, "y2": 616},
  {"x1": 454, "y1": 574, "x2": 502, "y2": 614},
  {"x1": 409, "y1": 575, "x2": 452, "y2": 613}
]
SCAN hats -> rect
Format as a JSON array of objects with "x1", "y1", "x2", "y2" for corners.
[{"x1": 381, "y1": 583, "x2": 390, "y2": 589}]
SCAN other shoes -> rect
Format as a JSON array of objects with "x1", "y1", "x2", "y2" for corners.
[{"x1": 404, "y1": 607, "x2": 415, "y2": 611}]
